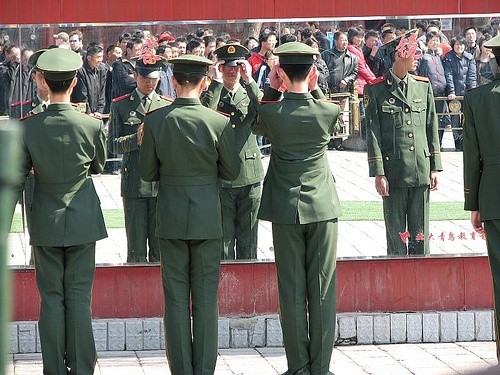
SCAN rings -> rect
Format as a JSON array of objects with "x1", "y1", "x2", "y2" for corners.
[{"x1": 473, "y1": 225, "x2": 476, "y2": 229}]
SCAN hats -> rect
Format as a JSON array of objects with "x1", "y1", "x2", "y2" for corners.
[
  {"x1": 131, "y1": 54, "x2": 167, "y2": 79},
  {"x1": 168, "y1": 54, "x2": 214, "y2": 73},
  {"x1": 213, "y1": 45, "x2": 251, "y2": 66},
  {"x1": 483, "y1": 35, "x2": 500, "y2": 49},
  {"x1": 37, "y1": 48, "x2": 83, "y2": 80},
  {"x1": 273, "y1": 42, "x2": 319, "y2": 64},
  {"x1": 27, "y1": 49, "x2": 47, "y2": 67},
  {"x1": 379, "y1": 29, "x2": 419, "y2": 52}
]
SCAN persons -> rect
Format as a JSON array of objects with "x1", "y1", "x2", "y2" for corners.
[
  {"x1": 0, "y1": 21, "x2": 500, "y2": 264},
  {"x1": 251, "y1": 41, "x2": 340, "y2": 375},
  {"x1": 139, "y1": 53, "x2": 243, "y2": 375},
  {"x1": 461, "y1": 35, "x2": 500, "y2": 358},
  {"x1": 0, "y1": 48, "x2": 106, "y2": 375}
]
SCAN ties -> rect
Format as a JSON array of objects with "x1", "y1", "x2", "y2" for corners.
[
  {"x1": 229, "y1": 91, "x2": 235, "y2": 100},
  {"x1": 142, "y1": 96, "x2": 150, "y2": 111},
  {"x1": 400, "y1": 81, "x2": 407, "y2": 97}
]
